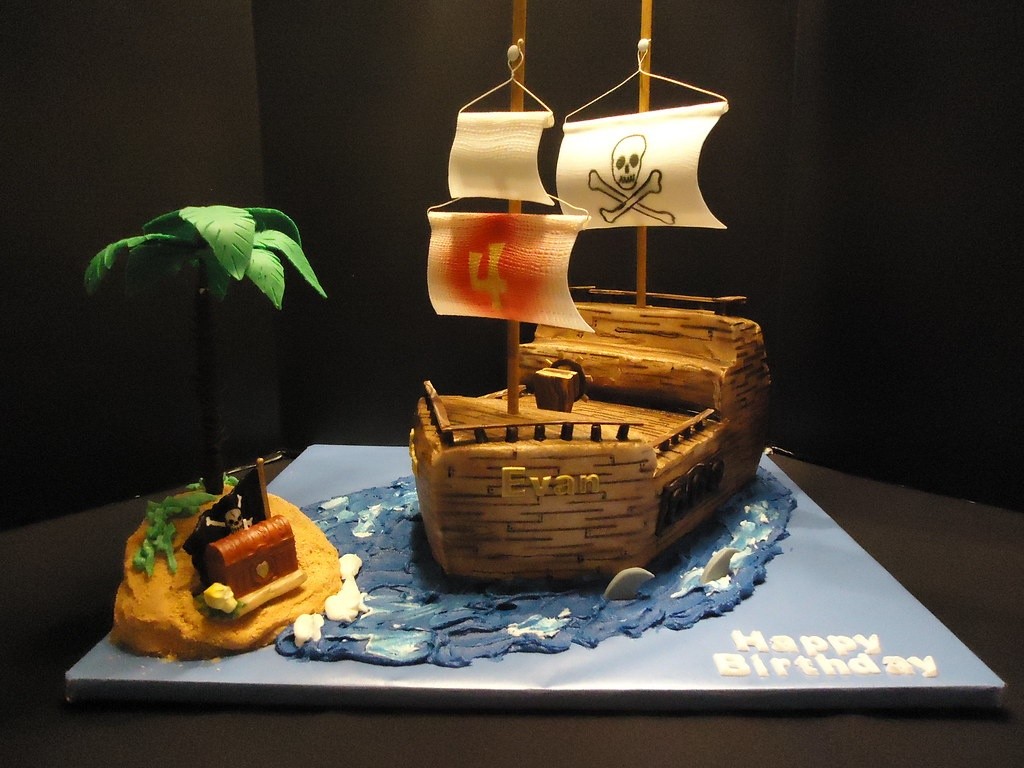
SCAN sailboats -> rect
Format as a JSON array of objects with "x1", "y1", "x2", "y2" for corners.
[{"x1": 403, "y1": 0, "x2": 774, "y2": 588}]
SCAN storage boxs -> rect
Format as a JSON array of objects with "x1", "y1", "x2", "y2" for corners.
[{"x1": 206, "y1": 513, "x2": 300, "y2": 598}]
